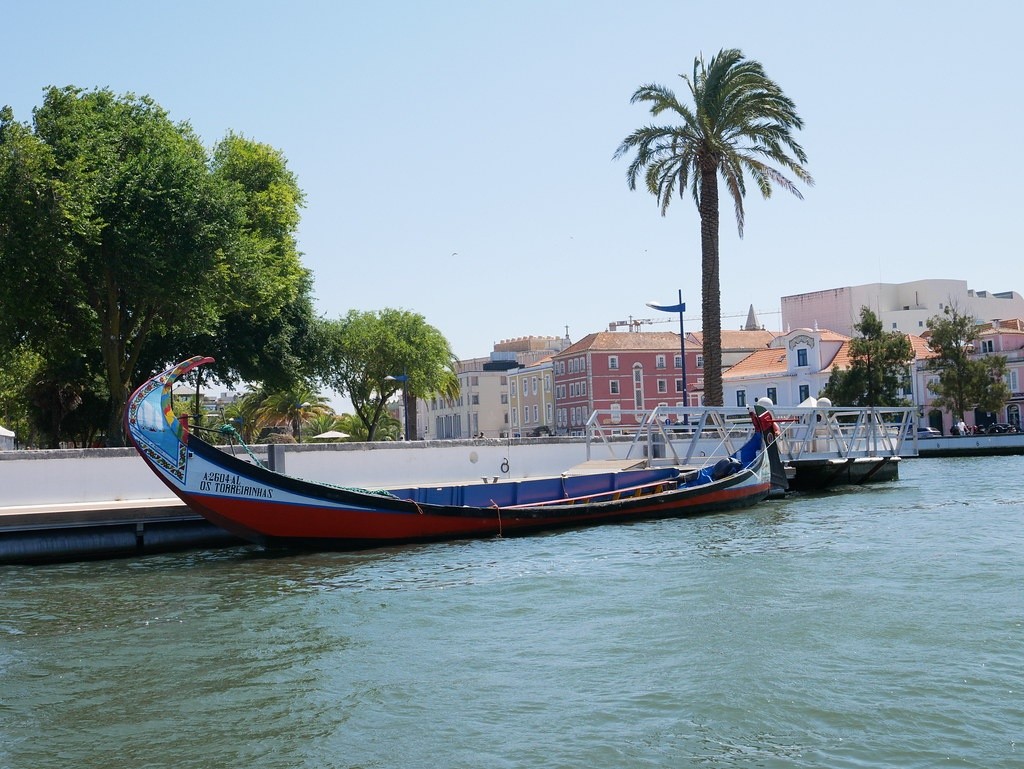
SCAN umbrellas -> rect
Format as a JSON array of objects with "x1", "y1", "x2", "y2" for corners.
[{"x1": 313, "y1": 430, "x2": 351, "y2": 439}]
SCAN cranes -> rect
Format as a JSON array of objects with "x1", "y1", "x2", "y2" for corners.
[{"x1": 609, "y1": 310, "x2": 781, "y2": 331}]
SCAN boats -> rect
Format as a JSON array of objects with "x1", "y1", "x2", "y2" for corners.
[{"x1": 127, "y1": 356, "x2": 796, "y2": 559}]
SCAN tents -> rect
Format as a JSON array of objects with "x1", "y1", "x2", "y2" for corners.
[{"x1": 0, "y1": 425, "x2": 15, "y2": 451}]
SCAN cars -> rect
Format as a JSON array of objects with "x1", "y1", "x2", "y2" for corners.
[
  {"x1": 906, "y1": 426, "x2": 942, "y2": 438},
  {"x1": 674, "y1": 415, "x2": 702, "y2": 433},
  {"x1": 950, "y1": 423, "x2": 1020, "y2": 435}
]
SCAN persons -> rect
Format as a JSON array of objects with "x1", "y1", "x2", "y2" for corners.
[
  {"x1": 479, "y1": 431, "x2": 487, "y2": 438},
  {"x1": 958, "y1": 419, "x2": 969, "y2": 436}
]
